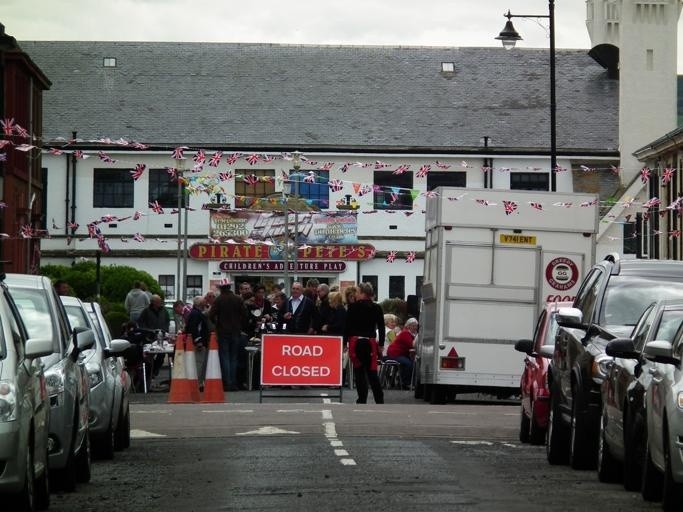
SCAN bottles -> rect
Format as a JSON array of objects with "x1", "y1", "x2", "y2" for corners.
[
  {"x1": 156, "y1": 332, "x2": 162, "y2": 347},
  {"x1": 168, "y1": 320, "x2": 176, "y2": 344},
  {"x1": 257, "y1": 320, "x2": 286, "y2": 338}
]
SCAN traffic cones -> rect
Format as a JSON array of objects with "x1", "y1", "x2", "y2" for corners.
[
  {"x1": 197, "y1": 331, "x2": 228, "y2": 402},
  {"x1": 166, "y1": 334, "x2": 194, "y2": 405},
  {"x1": 183, "y1": 331, "x2": 200, "y2": 404}
]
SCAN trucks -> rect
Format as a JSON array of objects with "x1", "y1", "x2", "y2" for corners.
[{"x1": 410, "y1": 185, "x2": 600, "y2": 405}]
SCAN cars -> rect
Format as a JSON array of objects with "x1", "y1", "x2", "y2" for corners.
[
  {"x1": 1, "y1": 271, "x2": 139, "y2": 510},
  {"x1": 158, "y1": 296, "x2": 194, "y2": 337}
]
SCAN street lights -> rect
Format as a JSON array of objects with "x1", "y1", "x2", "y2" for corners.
[
  {"x1": 172, "y1": 153, "x2": 186, "y2": 301},
  {"x1": 493, "y1": 2, "x2": 557, "y2": 191},
  {"x1": 291, "y1": 147, "x2": 303, "y2": 280},
  {"x1": 180, "y1": 174, "x2": 194, "y2": 302},
  {"x1": 280, "y1": 177, "x2": 290, "y2": 300}
]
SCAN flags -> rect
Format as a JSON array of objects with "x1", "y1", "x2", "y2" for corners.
[
  {"x1": 416, "y1": 160, "x2": 675, "y2": 190},
  {"x1": 419, "y1": 190, "x2": 682, "y2": 240},
  {"x1": 2, "y1": 117, "x2": 416, "y2": 265}
]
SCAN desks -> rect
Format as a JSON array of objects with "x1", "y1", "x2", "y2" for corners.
[
  {"x1": 243, "y1": 342, "x2": 261, "y2": 391},
  {"x1": 143, "y1": 345, "x2": 175, "y2": 393}
]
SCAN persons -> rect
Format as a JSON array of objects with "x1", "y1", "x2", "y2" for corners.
[
  {"x1": 119, "y1": 276, "x2": 421, "y2": 404},
  {"x1": 53, "y1": 280, "x2": 69, "y2": 296}
]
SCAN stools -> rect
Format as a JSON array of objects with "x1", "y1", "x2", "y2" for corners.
[{"x1": 376, "y1": 359, "x2": 404, "y2": 391}]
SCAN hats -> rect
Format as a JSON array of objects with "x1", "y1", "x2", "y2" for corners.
[
  {"x1": 216, "y1": 278, "x2": 233, "y2": 288},
  {"x1": 404, "y1": 318, "x2": 418, "y2": 327},
  {"x1": 384, "y1": 314, "x2": 397, "y2": 320}
]
